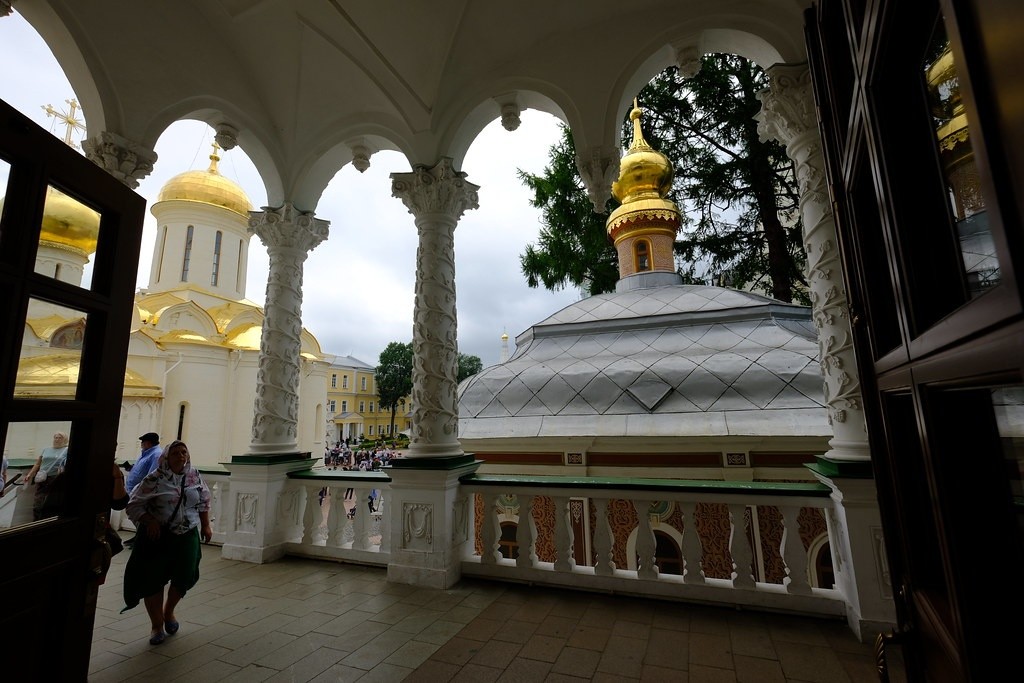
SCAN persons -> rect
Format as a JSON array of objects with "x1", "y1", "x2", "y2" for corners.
[
  {"x1": 1, "y1": 455, "x2": 8, "y2": 495},
  {"x1": 313, "y1": 431, "x2": 403, "y2": 515},
  {"x1": 43, "y1": 441, "x2": 131, "y2": 585},
  {"x1": 23, "y1": 432, "x2": 67, "y2": 523},
  {"x1": 124, "y1": 433, "x2": 164, "y2": 495},
  {"x1": 119, "y1": 440, "x2": 212, "y2": 644}
]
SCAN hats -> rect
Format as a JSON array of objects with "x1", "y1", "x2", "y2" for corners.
[{"x1": 139, "y1": 433, "x2": 159, "y2": 442}]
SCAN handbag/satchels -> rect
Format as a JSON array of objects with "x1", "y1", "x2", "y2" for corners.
[
  {"x1": 34, "y1": 469, "x2": 47, "y2": 482},
  {"x1": 133, "y1": 527, "x2": 178, "y2": 568}
]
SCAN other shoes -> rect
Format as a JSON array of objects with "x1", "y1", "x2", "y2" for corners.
[
  {"x1": 150, "y1": 631, "x2": 165, "y2": 645},
  {"x1": 164, "y1": 618, "x2": 179, "y2": 635}
]
